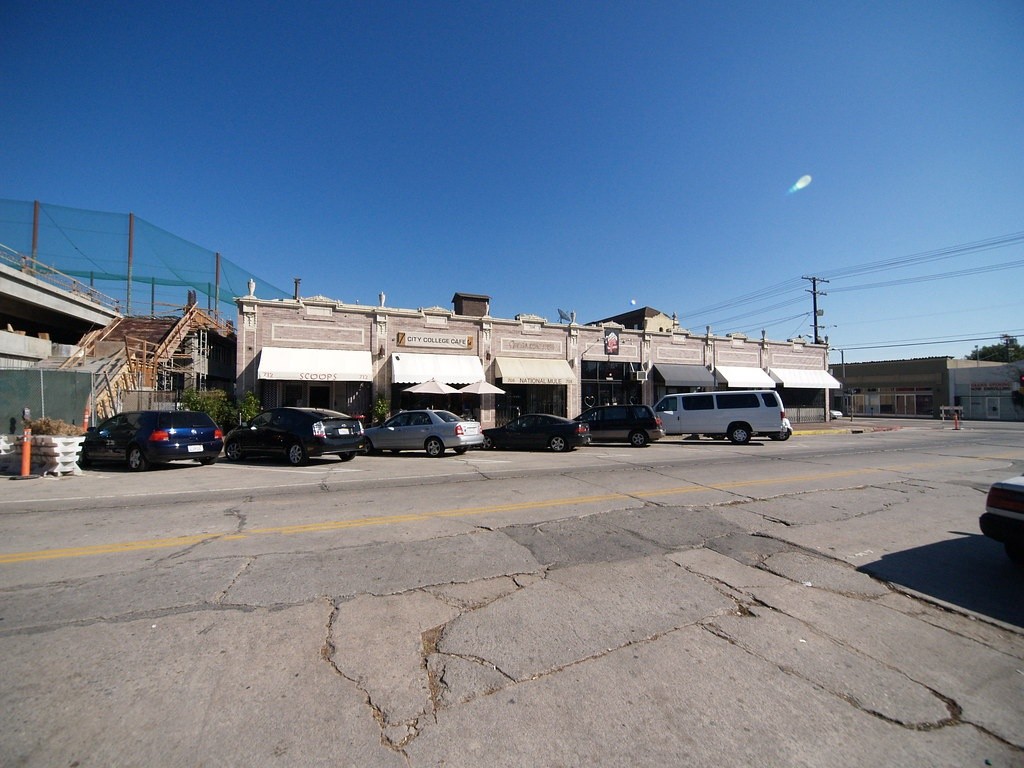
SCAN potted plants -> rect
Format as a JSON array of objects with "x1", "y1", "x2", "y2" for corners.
[
  {"x1": 370, "y1": 392, "x2": 391, "y2": 426},
  {"x1": 13, "y1": 417, "x2": 86, "y2": 477}
]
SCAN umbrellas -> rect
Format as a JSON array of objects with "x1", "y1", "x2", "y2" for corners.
[
  {"x1": 459, "y1": 379, "x2": 506, "y2": 395},
  {"x1": 402, "y1": 376, "x2": 463, "y2": 395}
]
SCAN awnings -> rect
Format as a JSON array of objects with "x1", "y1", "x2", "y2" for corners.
[
  {"x1": 391, "y1": 353, "x2": 486, "y2": 385},
  {"x1": 652, "y1": 363, "x2": 715, "y2": 387},
  {"x1": 494, "y1": 357, "x2": 577, "y2": 385},
  {"x1": 769, "y1": 368, "x2": 842, "y2": 389},
  {"x1": 257, "y1": 345, "x2": 374, "y2": 384},
  {"x1": 715, "y1": 366, "x2": 776, "y2": 388}
]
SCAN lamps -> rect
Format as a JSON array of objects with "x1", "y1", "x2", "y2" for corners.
[{"x1": 380, "y1": 344, "x2": 386, "y2": 358}]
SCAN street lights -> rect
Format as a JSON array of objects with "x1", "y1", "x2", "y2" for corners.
[{"x1": 832, "y1": 348, "x2": 846, "y2": 414}]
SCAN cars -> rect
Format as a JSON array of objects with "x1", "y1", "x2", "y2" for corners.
[
  {"x1": 225, "y1": 406, "x2": 366, "y2": 467},
  {"x1": 362, "y1": 408, "x2": 484, "y2": 458},
  {"x1": 829, "y1": 409, "x2": 843, "y2": 419},
  {"x1": 81, "y1": 409, "x2": 224, "y2": 471},
  {"x1": 979, "y1": 475, "x2": 1024, "y2": 564},
  {"x1": 480, "y1": 414, "x2": 593, "y2": 452}
]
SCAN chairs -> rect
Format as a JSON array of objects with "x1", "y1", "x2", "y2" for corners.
[{"x1": 414, "y1": 418, "x2": 425, "y2": 426}]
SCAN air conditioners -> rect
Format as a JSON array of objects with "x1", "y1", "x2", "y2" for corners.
[{"x1": 634, "y1": 371, "x2": 649, "y2": 382}]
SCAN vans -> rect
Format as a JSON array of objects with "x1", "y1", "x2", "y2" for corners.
[
  {"x1": 653, "y1": 390, "x2": 787, "y2": 446},
  {"x1": 569, "y1": 404, "x2": 666, "y2": 446}
]
